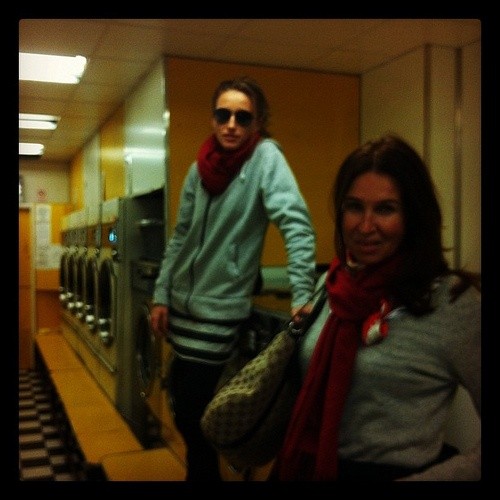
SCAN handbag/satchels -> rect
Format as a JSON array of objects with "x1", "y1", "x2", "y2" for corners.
[{"x1": 202, "y1": 271, "x2": 347, "y2": 476}]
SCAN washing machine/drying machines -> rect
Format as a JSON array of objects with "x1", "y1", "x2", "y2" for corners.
[
  {"x1": 129, "y1": 257, "x2": 162, "y2": 423},
  {"x1": 59, "y1": 220, "x2": 117, "y2": 407}
]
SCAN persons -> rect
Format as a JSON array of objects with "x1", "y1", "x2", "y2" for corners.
[
  {"x1": 148, "y1": 78, "x2": 321, "y2": 484},
  {"x1": 268, "y1": 133, "x2": 484, "y2": 482}
]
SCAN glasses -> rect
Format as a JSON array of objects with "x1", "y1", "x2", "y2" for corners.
[{"x1": 213, "y1": 107, "x2": 258, "y2": 129}]
